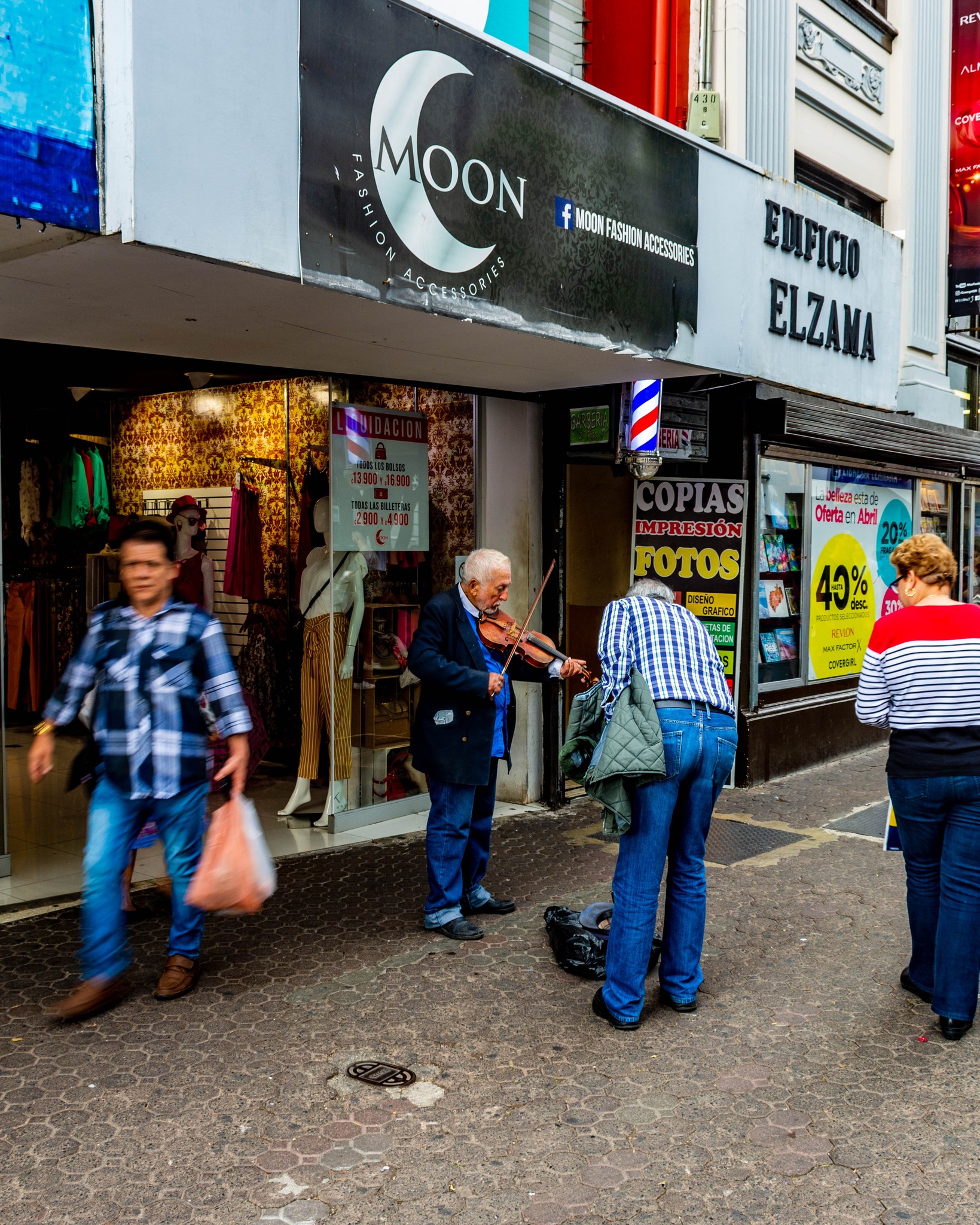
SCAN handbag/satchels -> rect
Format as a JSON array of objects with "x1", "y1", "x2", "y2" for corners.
[
  {"x1": 242, "y1": 796, "x2": 279, "y2": 902},
  {"x1": 184, "y1": 798, "x2": 261, "y2": 916},
  {"x1": 386, "y1": 753, "x2": 409, "y2": 801},
  {"x1": 374, "y1": 699, "x2": 410, "y2": 745},
  {"x1": 373, "y1": 634, "x2": 401, "y2": 670},
  {"x1": 285, "y1": 614, "x2": 305, "y2": 648}
]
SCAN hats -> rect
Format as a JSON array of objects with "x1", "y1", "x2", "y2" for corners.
[{"x1": 166, "y1": 495, "x2": 207, "y2": 525}]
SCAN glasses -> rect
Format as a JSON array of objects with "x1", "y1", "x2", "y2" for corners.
[
  {"x1": 890, "y1": 575, "x2": 906, "y2": 594},
  {"x1": 178, "y1": 514, "x2": 204, "y2": 527}
]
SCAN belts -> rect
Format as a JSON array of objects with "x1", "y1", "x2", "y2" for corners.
[{"x1": 654, "y1": 699, "x2": 727, "y2": 715}]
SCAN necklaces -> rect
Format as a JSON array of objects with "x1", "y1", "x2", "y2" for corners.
[{"x1": 926, "y1": 594, "x2": 950, "y2": 597}]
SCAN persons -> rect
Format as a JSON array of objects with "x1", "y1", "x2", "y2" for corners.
[
  {"x1": 27, "y1": 514, "x2": 254, "y2": 1024},
  {"x1": 854, "y1": 531, "x2": 980, "y2": 1041},
  {"x1": 408, "y1": 547, "x2": 586, "y2": 942},
  {"x1": 590, "y1": 579, "x2": 740, "y2": 1031},
  {"x1": 165, "y1": 495, "x2": 215, "y2": 619},
  {"x1": 277, "y1": 495, "x2": 368, "y2": 828},
  {"x1": 963, "y1": 544, "x2": 980, "y2": 605}
]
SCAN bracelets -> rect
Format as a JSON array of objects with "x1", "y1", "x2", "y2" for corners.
[{"x1": 33, "y1": 721, "x2": 53, "y2": 737}]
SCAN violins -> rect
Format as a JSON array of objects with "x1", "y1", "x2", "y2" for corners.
[{"x1": 478, "y1": 609, "x2": 600, "y2": 689}]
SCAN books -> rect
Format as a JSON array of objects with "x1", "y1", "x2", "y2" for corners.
[
  {"x1": 921, "y1": 487, "x2": 949, "y2": 512},
  {"x1": 760, "y1": 481, "x2": 800, "y2": 662},
  {"x1": 921, "y1": 515, "x2": 943, "y2": 536}
]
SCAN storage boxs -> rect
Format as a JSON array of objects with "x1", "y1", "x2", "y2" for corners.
[{"x1": 344, "y1": 603, "x2": 421, "y2": 811}]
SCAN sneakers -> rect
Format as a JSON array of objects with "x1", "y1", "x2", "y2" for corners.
[
  {"x1": 157, "y1": 954, "x2": 196, "y2": 999},
  {"x1": 50, "y1": 972, "x2": 126, "y2": 1020}
]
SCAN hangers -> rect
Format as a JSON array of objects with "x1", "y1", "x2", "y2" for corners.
[{"x1": 87, "y1": 436, "x2": 97, "y2": 449}]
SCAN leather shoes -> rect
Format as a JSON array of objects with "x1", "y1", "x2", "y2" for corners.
[
  {"x1": 463, "y1": 896, "x2": 516, "y2": 914},
  {"x1": 436, "y1": 917, "x2": 484, "y2": 939},
  {"x1": 592, "y1": 987, "x2": 641, "y2": 1029},
  {"x1": 661, "y1": 989, "x2": 698, "y2": 1009},
  {"x1": 940, "y1": 1015, "x2": 973, "y2": 1041},
  {"x1": 900, "y1": 966, "x2": 933, "y2": 1003}
]
